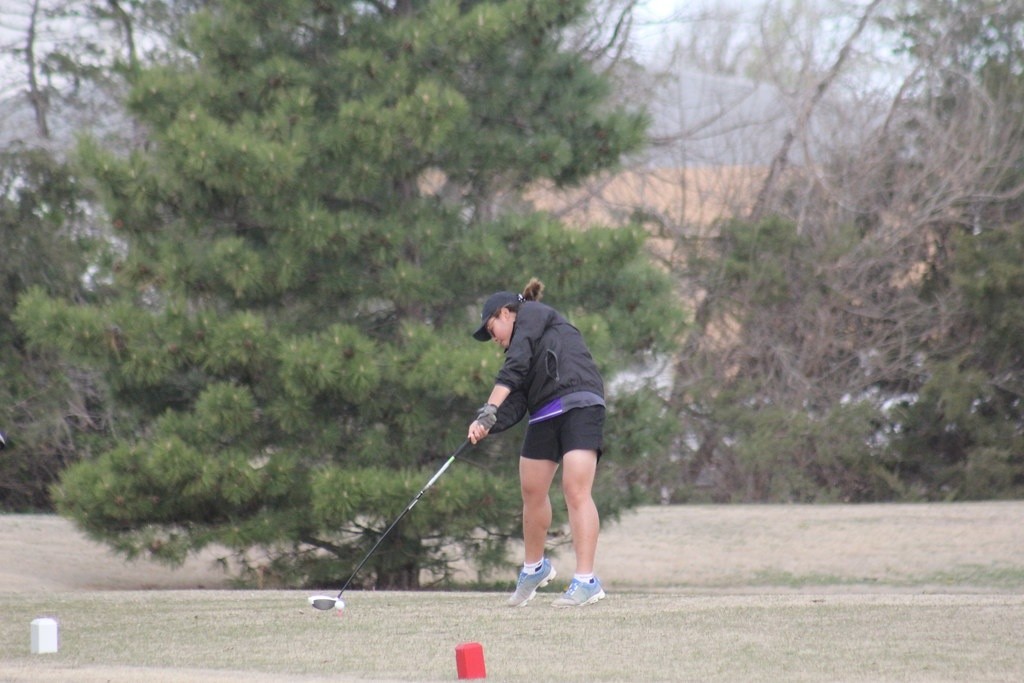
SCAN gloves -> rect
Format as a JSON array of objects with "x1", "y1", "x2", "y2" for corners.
[{"x1": 476, "y1": 403, "x2": 498, "y2": 430}]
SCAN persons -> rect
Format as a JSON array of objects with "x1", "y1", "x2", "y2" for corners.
[{"x1": 467, "y1": 279, "x2": 606, "y2": 607}]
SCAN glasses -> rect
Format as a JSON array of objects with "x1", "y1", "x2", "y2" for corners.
[{"x1": 486, "y1": 317, "x2": 497, "y2": 337}]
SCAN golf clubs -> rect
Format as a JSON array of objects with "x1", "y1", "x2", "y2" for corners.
[{"x1": 307, "y1": 406, "x2": 497, "y2": 611}]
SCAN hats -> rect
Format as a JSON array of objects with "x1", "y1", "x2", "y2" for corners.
[{"x1": 472, "y1": 291, "x2": 523, "y2": 342}]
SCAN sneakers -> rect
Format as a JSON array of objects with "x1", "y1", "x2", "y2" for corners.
[
  {"x1": 550, "y1": 576, "x2": 605, "y2": 609},
  {"x1": 506, "y1": 556, "x2": 557, "y2": 608}
]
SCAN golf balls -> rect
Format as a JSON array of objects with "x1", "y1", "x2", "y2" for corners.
[{"x1": 334, "y1": 601, "x2": 344, "y2": 610}]
